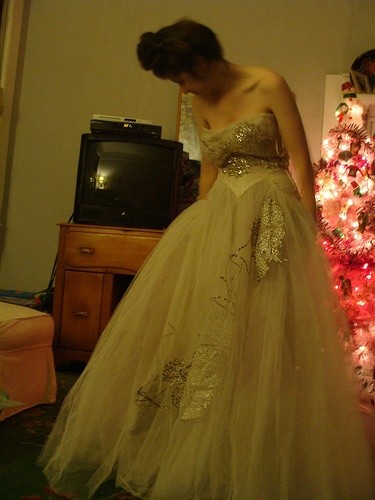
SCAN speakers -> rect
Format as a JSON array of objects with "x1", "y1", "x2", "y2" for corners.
[{"x1": 174, "y1": 159, "x2": 201, "y2": 202}]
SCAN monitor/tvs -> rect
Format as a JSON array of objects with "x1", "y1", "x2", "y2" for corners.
[{"x1": 73, "y1": 133, "x2": 184, "y2": 232}]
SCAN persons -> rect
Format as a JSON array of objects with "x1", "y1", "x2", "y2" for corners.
[{"x1": 32, "y1": 19, "x2": 375, "y2": 499}]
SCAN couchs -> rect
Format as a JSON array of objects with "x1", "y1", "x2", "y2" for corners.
[{"x1": 0, "y1": 300, "x2": 59, "y2": 424}]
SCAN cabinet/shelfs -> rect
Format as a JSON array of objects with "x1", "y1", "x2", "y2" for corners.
[{"x1": 50, "y1": 222, "x2": 165, "y2": 374}]
